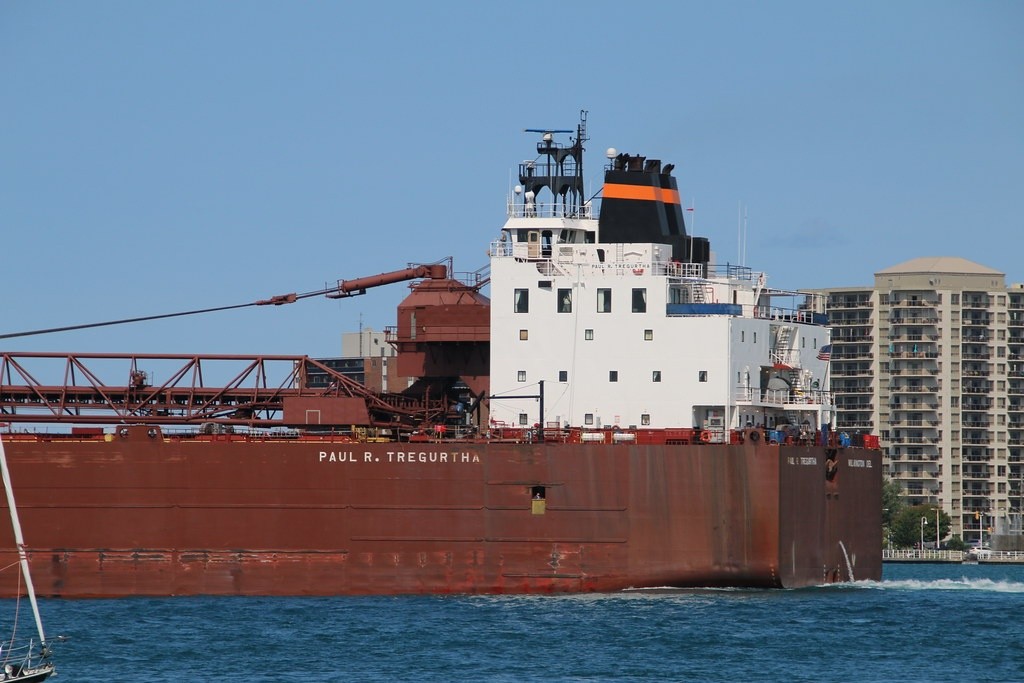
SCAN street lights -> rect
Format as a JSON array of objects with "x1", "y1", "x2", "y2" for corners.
[{"x1": 921, "y1": 516, "x2": 927, "y2": 551}]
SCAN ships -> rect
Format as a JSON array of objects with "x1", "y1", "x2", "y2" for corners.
[{"x1": 0, "y1": 108, "x2": 892, "y2": 593}]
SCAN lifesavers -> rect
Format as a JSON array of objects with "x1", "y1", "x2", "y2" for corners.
[{"x1": 700, "y1": 431, "x2": 712, "y2": 442}]
